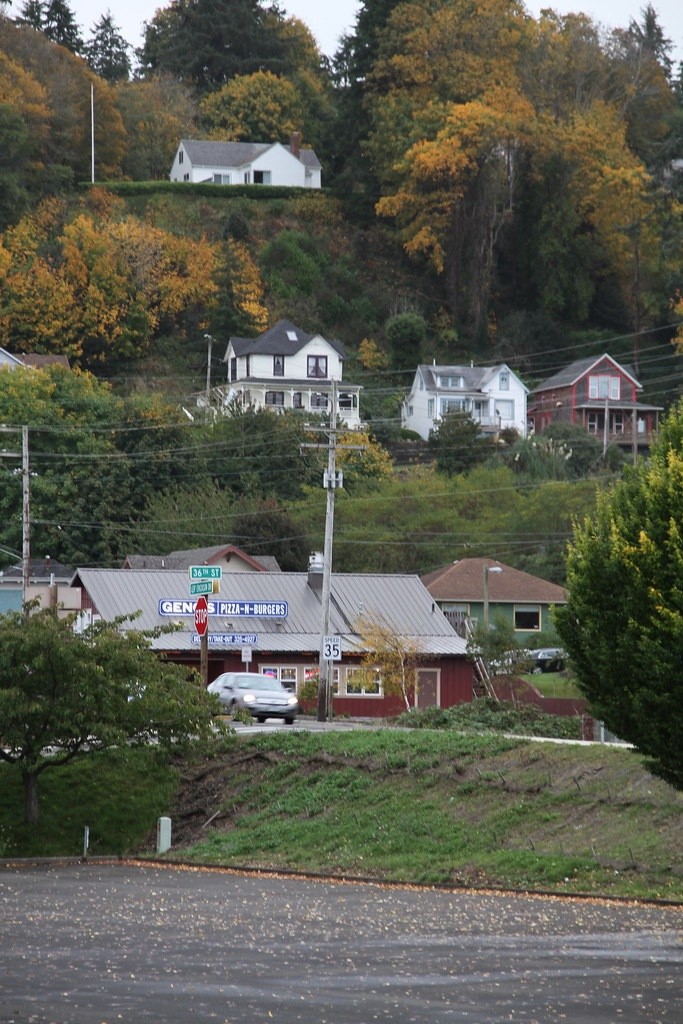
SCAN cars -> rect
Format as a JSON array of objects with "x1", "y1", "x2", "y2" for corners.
[
  {"x1": 490, "y1": 648, "x2": 568, "y2": 675},
  {"x1": 207, "y1": 672, "x2": 299, "y2": 724}
]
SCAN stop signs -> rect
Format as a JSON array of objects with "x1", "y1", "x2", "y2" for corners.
[{"x1": 194, "y1": 596, "x2": 209, "y2": 637}]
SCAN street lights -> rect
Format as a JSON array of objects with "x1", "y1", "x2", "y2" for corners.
[{"x1": 483, "y1": 561, "x2": 503, "y2": 631}]
